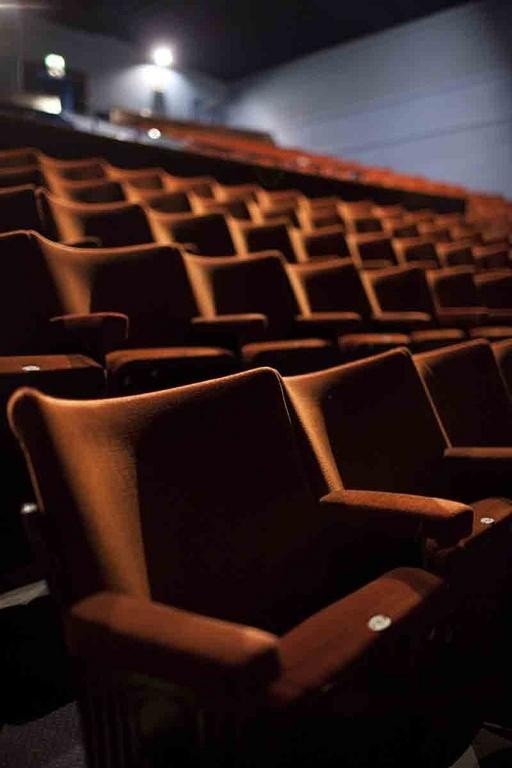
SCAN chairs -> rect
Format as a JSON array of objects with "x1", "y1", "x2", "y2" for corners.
[{"x1": 0, "y1": 143, "x2": 510, "y2": 767}]
